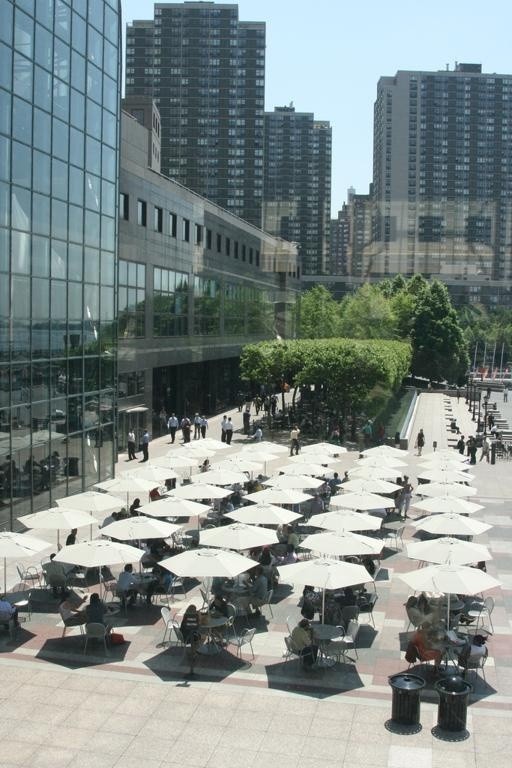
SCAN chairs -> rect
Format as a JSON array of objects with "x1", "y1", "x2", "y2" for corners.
[{"x1": 0, "y1": 475, "x2": 494, "y2": 692}]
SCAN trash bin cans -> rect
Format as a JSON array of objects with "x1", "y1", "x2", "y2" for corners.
[
  {"x1": 388, "y1": 673, "x2": 426, "y2": 724},
  {"x1": 436, "y1": 679, "x2": 473, "y2": 732}
]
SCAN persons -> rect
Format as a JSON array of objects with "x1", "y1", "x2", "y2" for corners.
[
  {"x1": 412, "y1": 622, "x2": 443, "y2": 679},
  {"x1": 458, "y1": 634, "x2": 488, "y2": 669},
  {"x1": 127, "y1": 427, "x2": 139, "y2": 460},
  {"x1": 449, "y1": 412, "x2": 499, "y2": 466},
  {"x1": 141, "y1": 429, "x2": 150, "y2": 462},
  {"x1": 414, "y1": 428, "x2": 426, "y2": 457},
  {"x1": 361, "y1": 419, "x2": 374, "y2": 449},
  {"x1": 179, "y1": 603, "x2": 203, "y2": 658},
  {"x1": 486, "y1": 386, "x2": 491, "y2": 399},
  {"x1": 455, "y1": 388, "x2": 461, "y2": 404},
  {"x1": 502, "y1": 387, "x2": 508, "y2": 402},
  {"x1": 289, "y1": 425, "x2": 301, "y2": 456},
  {"x1": 165, "y1": 391, "x2": 279, "y2": 446},
  {"x1": 290, "y1": 617, "x2": 320, "y2": 672}
]
